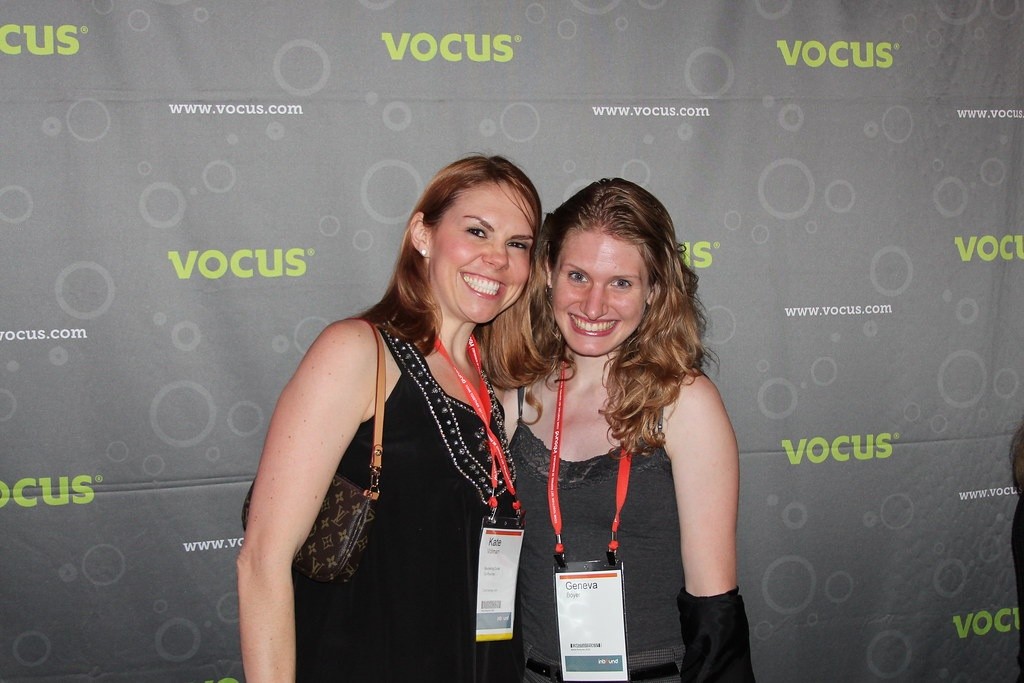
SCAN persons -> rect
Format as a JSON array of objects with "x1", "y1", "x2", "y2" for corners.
[
  {"x1": 234, "y1": 150, "x2": 550, "y2": 683},
  {"x1": 504, "y1": 174, "x2": 754, "y2": 683}
]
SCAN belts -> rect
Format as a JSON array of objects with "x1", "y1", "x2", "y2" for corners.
[{"x1": 526, "y1": 658, "x2": 679, "y2": 683}]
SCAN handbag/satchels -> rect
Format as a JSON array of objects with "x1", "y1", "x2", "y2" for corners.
[{"x1": 242, "y1": 318, "x2": 386, "y2": 584}]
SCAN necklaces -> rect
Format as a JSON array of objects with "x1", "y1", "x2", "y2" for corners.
[{"x1": 383, "y1": 321, "x2": 518, "y2": 505}]
